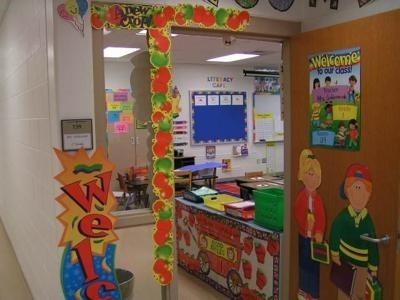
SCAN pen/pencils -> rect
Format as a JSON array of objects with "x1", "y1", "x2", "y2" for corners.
[{"x1": 259, "y1": 139, "x2": 266, "y2": 142}]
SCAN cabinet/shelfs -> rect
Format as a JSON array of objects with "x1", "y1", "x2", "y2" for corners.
[{"x1": 105, "y1": 86, "x2": 154, "y2": 193}]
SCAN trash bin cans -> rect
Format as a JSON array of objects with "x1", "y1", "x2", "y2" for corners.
[{"x1": 115, "y1": 268, "x2": 135, "y2": 300}]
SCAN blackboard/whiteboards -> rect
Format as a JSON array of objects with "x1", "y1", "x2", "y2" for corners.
[
  {"x1": 191, "y1": 89, "x2": 248, "y2": 144},
  {"x1": 253, "y1": 91, "x2": 284, "y2": 143}
]
